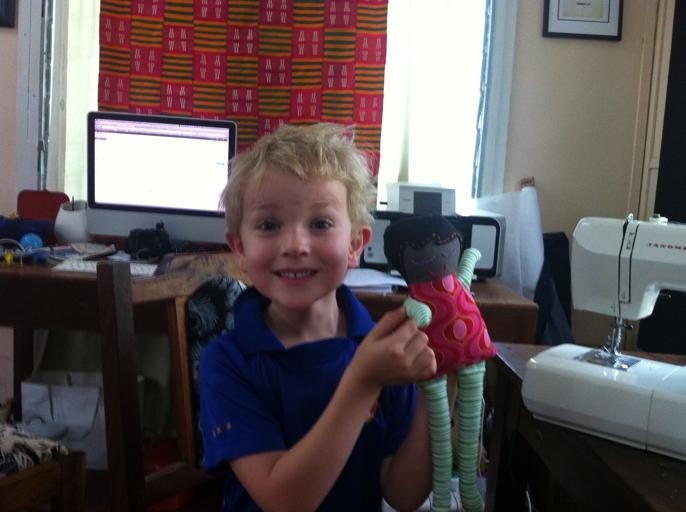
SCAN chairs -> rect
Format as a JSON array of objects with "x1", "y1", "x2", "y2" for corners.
[
  {"x1": 94, "y1": 256, "x2": 240, "y2": 511},
  {"x1": 0, "y1": 448, "x2": 86, "y2": 512}
]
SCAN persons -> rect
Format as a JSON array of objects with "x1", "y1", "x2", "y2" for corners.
[{"x1": 197, "y1": 122, "x2": 457, "y2": 511}]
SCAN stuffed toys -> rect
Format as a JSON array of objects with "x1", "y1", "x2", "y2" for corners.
[{"x1": 384, "y1": 214, "x2": 497, "y2": 511}]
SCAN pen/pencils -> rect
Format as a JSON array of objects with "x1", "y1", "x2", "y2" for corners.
[
  {"x1": 72, "y1": 195, "x2": 74, "y2": 212},
  {"x1": 84, "y1": 201, "x2": 88, "y2": 210}
]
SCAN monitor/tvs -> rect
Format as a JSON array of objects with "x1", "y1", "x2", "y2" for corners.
[{"x1": 85, "y1": 111, "x2": 238, "y2": 245}]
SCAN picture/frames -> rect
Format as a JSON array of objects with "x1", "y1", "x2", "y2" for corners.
[{"x1": 539, "y1": 0, "x2": 625, "y2": 41}]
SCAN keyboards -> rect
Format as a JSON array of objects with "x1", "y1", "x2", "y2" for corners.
[{"x1": 54, "y1": 257, "x2": 159, "y2": 277}]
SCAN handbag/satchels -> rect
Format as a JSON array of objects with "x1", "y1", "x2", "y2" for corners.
[{"x1": 17, "y1": 365, "x2": 147, "y2": 472}]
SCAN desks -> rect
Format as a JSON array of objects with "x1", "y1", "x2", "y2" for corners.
[
  {"x1": 483, "y1": 338, "x2": 686, "y2": 511},
  {"x1": 0, "y1": 248, "x2": 230, "y2": 426},
  {"x1": 342, "y1": 271, "x2": 540, "y2": 346}
]
